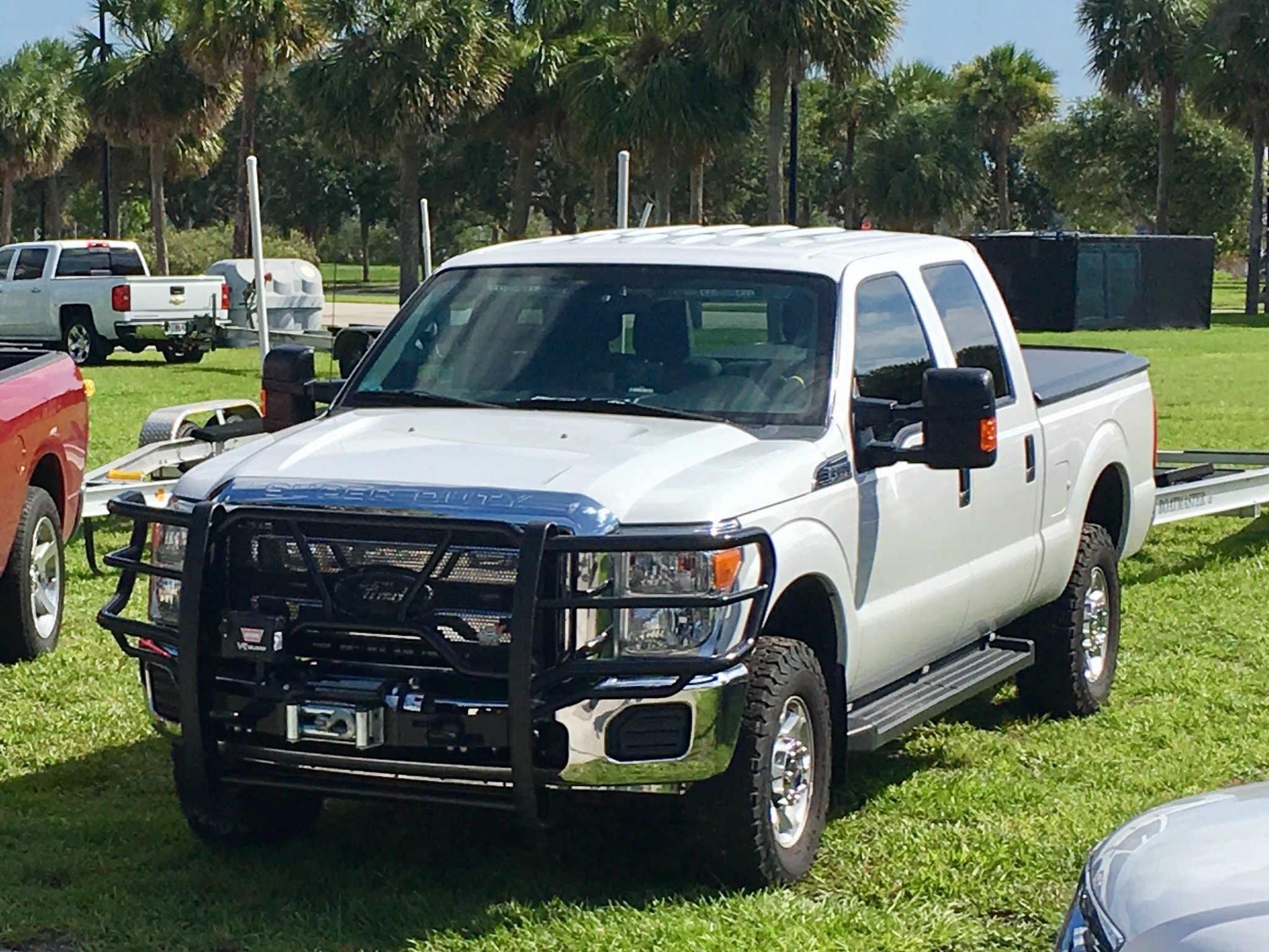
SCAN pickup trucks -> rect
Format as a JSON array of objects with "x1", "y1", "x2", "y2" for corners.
[
  {"x1": 0, "y1": 351, "x2": 87, "y2": 665},
  {"x1": 98, "y1": 224, "x2": 1155, "y2": 888},
  {"x1": 0, "y1": 240, "x2": 232, "y2": 366}
]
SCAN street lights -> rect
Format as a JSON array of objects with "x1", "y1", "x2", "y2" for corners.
[{"x1": 785, "y1": 37, "x2": 810, "y2": 221}]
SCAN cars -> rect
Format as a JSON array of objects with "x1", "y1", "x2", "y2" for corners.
[{"x1": 1052, "y1": 778, "x2": 1267, "y2": 952}]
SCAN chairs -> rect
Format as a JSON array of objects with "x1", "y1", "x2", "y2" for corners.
[{"x1": 535, "y1": 290, "x2": 827, "y2": 429}]
